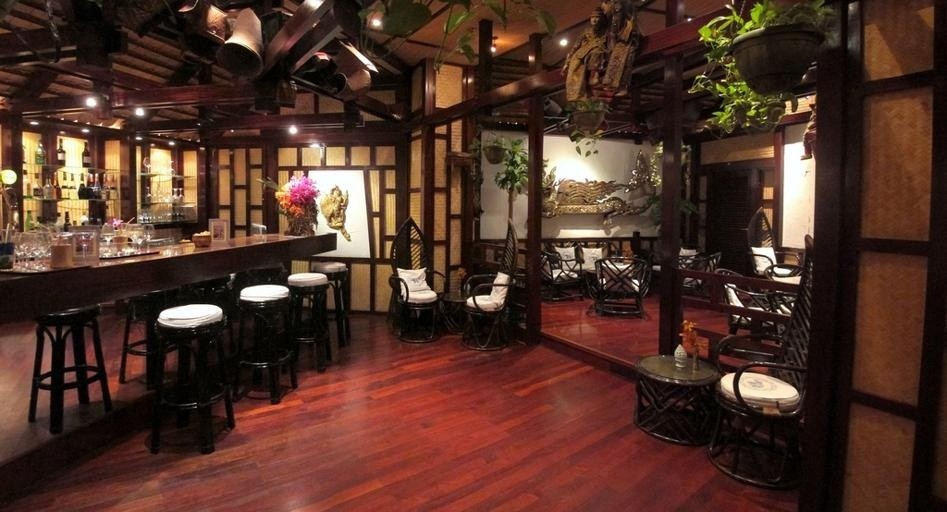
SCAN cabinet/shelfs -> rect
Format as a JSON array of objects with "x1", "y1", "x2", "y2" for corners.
[
  {"x1": 134, "y1": 171, "x2": 196, "y2": 205},
  {"x1": 19, "y1": 161, "x2": 119, "y2": 202}
]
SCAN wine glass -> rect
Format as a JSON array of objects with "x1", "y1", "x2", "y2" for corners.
[
  {"x1": 142, "y1": 206, "x2": 190, "y2": 223},
  {"x1": 12, "y1": 227, "x2": 55, "y2": 270},
  {"x1": 75, "y1": 223, "x2": 155, "y2": 266},
  {"x1": 142, "y1": 157, "x2": 176, "y2": 174},
  {"x1": 143, "y1": 186, "x2": 185, "y2": 205}
]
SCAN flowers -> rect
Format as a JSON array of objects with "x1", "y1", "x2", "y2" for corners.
[{"x1": 249, "y1": 170, "x2": 321, "y2": 239}]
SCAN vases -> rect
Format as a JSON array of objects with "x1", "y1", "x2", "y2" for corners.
[{"x1": 279, "y1": 201, "x2": 318, "y2": 238}]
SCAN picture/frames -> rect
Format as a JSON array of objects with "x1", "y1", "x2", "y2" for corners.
[{"x1": 207, "y1": 218, "x2": 229, "y2": 242}]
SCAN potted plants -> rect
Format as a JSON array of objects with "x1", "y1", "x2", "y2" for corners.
[
  {"x1": 686, "y1": 1, "x2": 839, "y2": 142},
  {"x1": 561, "y1": 98, "x2": 615, "y2": 159},
  {"x1": 464, "y1": 113, "x2": 551, "y2": 195}
]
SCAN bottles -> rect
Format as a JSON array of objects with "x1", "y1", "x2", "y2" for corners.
[
  {"x1": 78, "y1": 173, "x2": 86, "y2": 199},
  {"x1": 32, "y1": 173, "x2": 43, "y2": 200},
  {"x1": 110, "y1": 174, "x2": 119, "y2": 201},
  {"x1": 86, "y1": 173, "x2": 94, "y2": 199},
  {"x1": 23, "y1": 169, "x2": 31, "y2": 199},
  {"x1": 69, "y1": 173, "x2": 78, "y2": 199},
  {"x1": 93, "y1": 174, "x2": 102, "y2": 199},
  {"x1": 100, "y1": 174, "x2": 109, "y2": 199},
  {"x1": 55, "y1": 138, "x2": 65, "y2": 166},
  {"x1": 35, "y1": 139, "x2": 47, "y2": 164},
  {"x1": 23, "y1": 210, "x2": 102, "y2": 233},
  {"x1": 81, "y1": 141, "x2": 92, "y2": 166},
  {"x1": 53, "y1": 171, "x2": 62, "y2": 200},
  {"x1": 43, "y1": 173, "x2": 56, "y2": 200},
  {"x1": 62, "y1": 172, "x2": 69, "y2": 198}
]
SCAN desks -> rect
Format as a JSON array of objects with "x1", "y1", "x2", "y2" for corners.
[{"x1": 1, "y1": 234, "x2": 339, "y2": 327}]
